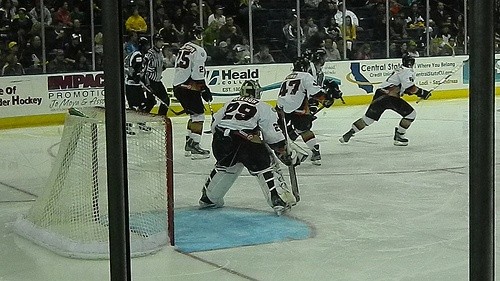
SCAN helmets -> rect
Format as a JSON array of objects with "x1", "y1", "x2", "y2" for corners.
[
  {"x1": 138, "y1": 37, "x2": 149, "y2": 54},
  {"x1": 190, "y1": 26, "x2": 203, "y2": 42},
  {"x1": 402, "y1": 50, "x2": 419, "y2": 67},
  {"x1": 292, "y1": 57, "x2": 311, "y2": 72},
  {"x1": 152, "y1": 32, "x2": 165, "y2": 53},
  {"x1": 310, "y1": 48, "x2": 326, "y2": 65}
]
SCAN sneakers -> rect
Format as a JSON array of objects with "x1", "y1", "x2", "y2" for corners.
[
  {"x1": 184, "y1": 135, "x2": 193, "y2": 157},
  {"x1": 310, "y1": 144, "x2": 322, "y2": 164},
  {"x1": 198, "y1": 197, "x2": 224, "y2": 209},
  {"x1": 139, "y1": 124, "x2": 152, "y2": 134},
  {"x1": 273, "y1": 203, "x2": 284, "y2": 216},
  {"x1": 339, "y1": 132, "x2": 355, "y2": 144},
  {"x1": 393, "y1": 127, "x2": 411, "y2": 146},
  {"x1": 190, "y1": 141, "x2": 210, "y2": 159},
  {"x1": 126, "y1": 126, "x2": 136, "y2": 138}
]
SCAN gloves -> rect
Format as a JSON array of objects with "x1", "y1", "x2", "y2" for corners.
[
  {"x1": 278, "y1": 149, "x2": 300, "y2": 165},
  {"x1": 201, "y1": 89, "x2": 213, "y2": 102},
  {"x1": 321, "y1": 96, "x2": 335, "y2": 108},
  {"x1": 417, "y1": 89, "x2": 432, "y2": 99},
  {"x1": 330, "y1": 87, "x2": 343, "y2": 99}
]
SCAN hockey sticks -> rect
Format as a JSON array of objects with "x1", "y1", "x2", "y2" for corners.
[
  {"x1": 208, "y1": 101, "x2": 215, "y2": 125},
  {"x1": 139, "y1": 79, "x2": 185, "y2": 115},
  {"x1": 311, "y1": 105, "x2": 324, "y2": 115},
  {"x1": 280, "y1": 110, "x2": 301, "y2": 202},
  {"x1": 416, "y1": 57, "x2": 469, "y2": 104}
]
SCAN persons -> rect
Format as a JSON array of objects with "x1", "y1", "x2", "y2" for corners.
[
  {"x1": 174, "y1": 27, "x2": 213, "y2": 154},
  {"x1": 343, "y1": 54, "x2": 431, "y2": 142},
  {"x1": 124, "y1": 34, "x2": 170, "y2": 135},
  {"x1": 276, "y1": 49, "x2": 345, "y2": 161},
  {"x1": 0, "y1": 0, "x2": 470, "y2": 76},
  {"x1": 200, "y1": 80, "x2": 296, "y2": 206}
]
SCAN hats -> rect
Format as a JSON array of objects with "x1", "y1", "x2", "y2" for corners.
[{"x1": 9, "y1": 41, "x2": 17, "y2": 48}]
